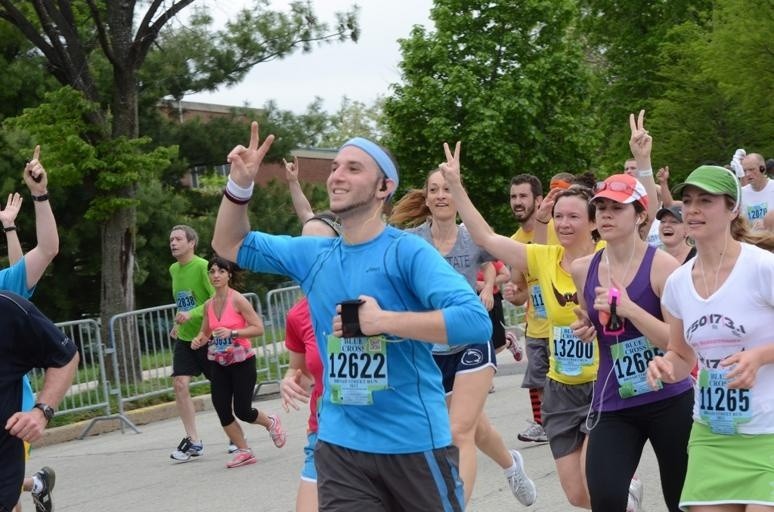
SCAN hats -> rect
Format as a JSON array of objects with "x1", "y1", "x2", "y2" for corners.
[
  {"x1": 669, "y1": 163, "x2": 742, "y2": 212},
  {"x1": 655, "y1": 203, "x2": 682, "y2": 221},
  {"x1": 588, "y1": 171, "x2": 653, "y2": 218}
]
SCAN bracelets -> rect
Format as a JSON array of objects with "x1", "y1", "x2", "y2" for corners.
[
  {"x1": 223, "y1": 174, "x2": 255, "y2": 206},
  {"x1": 3, "y1": 226, "x2": 16, "y2": 234},
  {"x1": 29, "y1": 191, "x2": 48, "y2": 203},
  {"x1": 635, "y1": 166, "x2": 652, "y2": 178},
  {"x1": 230, "y1": 329, "x2": 238, "y2": 339}
]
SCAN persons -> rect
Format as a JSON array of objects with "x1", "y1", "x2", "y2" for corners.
[
  {"x1": 389, "y1": 164, "x2": 540, "y2": 511},
  {"x1": 164, "y1": 217, "x2": 218, "y2": 466},
  {"x1": 274, "y1": 152, "x2": 316, "y2": 225},
  {"x1": 0, "y1": 190, "x2": 26, "y2": 269},
  {"x1": 182, "y1": 255, "x2": 292, "y2": 472},
  {"x1": 207, "y1": 116, "x2": 498, "y2": 511},
  {"x1": 436, "y1": 107, "x2": 658, "y2": 511},
  {"x1": 278, "y1": 209, "x2": 344, "y2": 512},
  {"x1": 0, "y1": 140, "x2": 61, "y2": 512},
  {"x1": 0, "y1": 289, "x2": 81, "y2": 512}
]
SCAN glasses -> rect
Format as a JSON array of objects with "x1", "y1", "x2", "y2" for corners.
[{"x1": 594, "y1": 177, "x2": 647, "y2": 207}]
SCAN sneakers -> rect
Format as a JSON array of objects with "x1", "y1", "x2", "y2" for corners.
[
  {"x1": 503, "y1": 446, "x2": 541, "y2": 508},
  {"x1": 516, "y1": 419, "x2": 552, "y2": 443},
  {"x1": 168, "y1": 437, "x2": 209, "y2": 462},
  {"x1": 224, "y1": 447, "x2": 260, "y2": 472},
  {"x1": 29, "y1": 465, "x2": 58, "y2": 512},
  {"x1": 265, "y1": 413, "x2": 289, "y2": 451},
  {"x1": 503, "y1": 330, "x2": 526, "y2": 363}
]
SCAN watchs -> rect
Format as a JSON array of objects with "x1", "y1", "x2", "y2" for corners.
[{"x1": 32, "y1": 401, "x2": 54, "y2": 421}]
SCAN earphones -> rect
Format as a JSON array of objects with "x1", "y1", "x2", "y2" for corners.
[
  {"x1": 379, "y1": 178, "x2": 387, "y2": 191},
  {"x1": 760, "y1": 165, "x2": 765, "y2": 173},
  {"x1": 635, "y1": 216, "x2": 641, "y2": 225}
]
